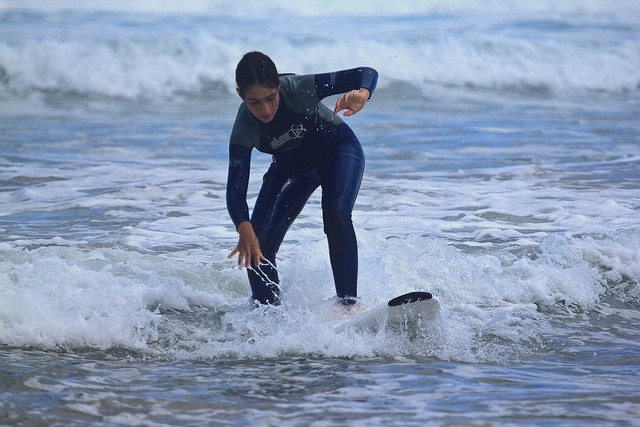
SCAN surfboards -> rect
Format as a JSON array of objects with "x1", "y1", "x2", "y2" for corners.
[{"x1": 332, "y1": 291, "x2": 442, "y2": 337}]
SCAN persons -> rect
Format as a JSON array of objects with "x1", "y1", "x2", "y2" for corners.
[{"x1": 225, "y1": 51, "x2": 377, "y2": 308}]
